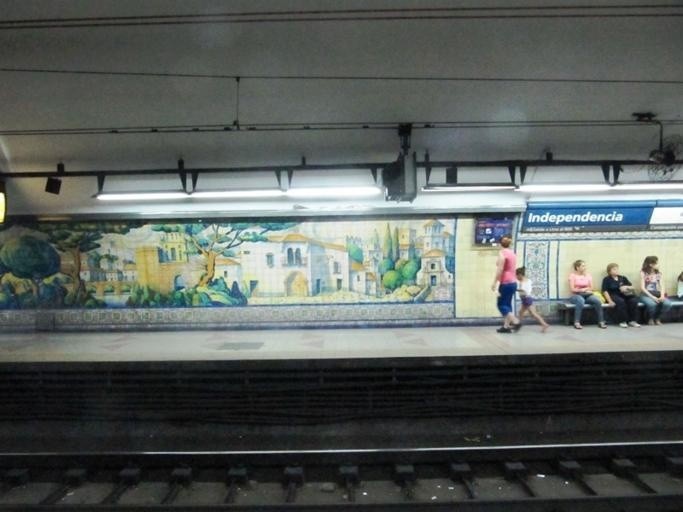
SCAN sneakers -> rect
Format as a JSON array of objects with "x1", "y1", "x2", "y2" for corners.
[
  {"x1": 597, "y1": 322, "x2": 607, "y2": 329},
  {"x1": 574, "y1": 322, "x2": 582, "y2": 330},
  {"x1": 495, "y1": 322, "x2": 521, "y2": 335},
  {"x1": 619, "y1": 320, "x2": 640, "y2": 329}
]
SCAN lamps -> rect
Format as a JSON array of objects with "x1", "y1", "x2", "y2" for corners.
[
  {"x1": 420, "y1": 168, "x2": 518, "y2": 193},
  {"x1": 91, "y1": 169, "x2": 189, "y2": 201},
  {"x1": 516, "y1": 166, "x2": 612, "y2": 192},
  {"x1": 611, "y1": 169, "x2": 682, "y2": 191},
  {"x1": 45, "y1": 162, "x2": 66, "y2": 195},
  {"x1": 286, "y1": 169, "x2": 382, "y2": 199},
  {"x1": 188, "y1": 169, "x2": 285, "y2": 200}
]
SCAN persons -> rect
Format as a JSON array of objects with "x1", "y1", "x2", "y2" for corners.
[
  {"x1": 489, "y1": 237, "x2": 522, "y2": 334},
  {"x1": 675, "y1": 271, "x2": 683, "y2": 301},
  {"x1": 601, "y1": 262, "x2": 641, "y2": 328},
  {"x1": 639, "y1": 256, "x2": 665, "y2": 326},
  {"x1": 514, "y1": 266, "x2": 550, "y2": 334},
  {"x1": 569, "y1": 259, "x2": 607, "y2": 329}
]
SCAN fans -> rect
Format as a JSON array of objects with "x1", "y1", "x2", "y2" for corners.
[{"x1": 632, "y1": 112, "x2": 681, "y2": 181}]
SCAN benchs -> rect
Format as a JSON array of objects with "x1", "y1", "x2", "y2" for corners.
[{"x1": 557, "y1": 295, "x2": 682, "y2": 325}]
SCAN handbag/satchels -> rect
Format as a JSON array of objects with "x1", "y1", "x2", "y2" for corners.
[{"x1": 591, "y1": 288, "x2": 606, "y2": 305}]
